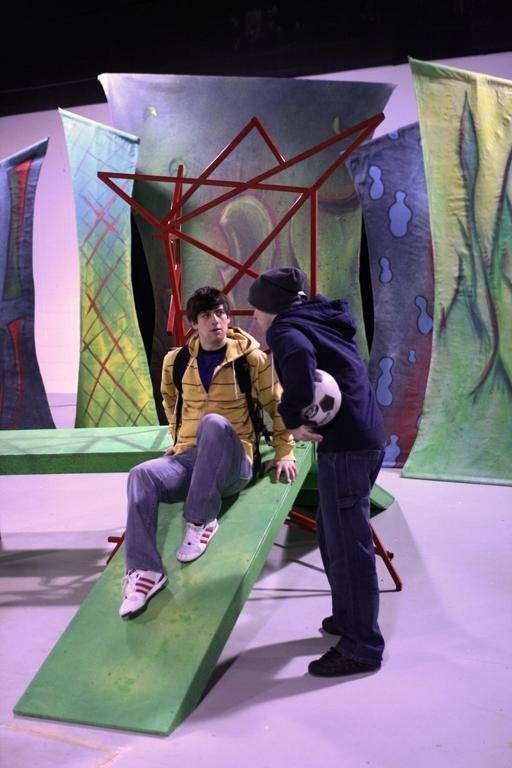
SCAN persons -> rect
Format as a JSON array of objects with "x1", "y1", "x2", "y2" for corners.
[
  {"x1": 118, "y1": 287, "x2": 299, "y2": 617},
  {"x1": 247, "y1": 267, "x2": 387, "y2": 677}
]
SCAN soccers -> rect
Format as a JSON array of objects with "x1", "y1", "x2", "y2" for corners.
[{"x1": 301, "y1": 368, "x2": 342, "y2": 424}]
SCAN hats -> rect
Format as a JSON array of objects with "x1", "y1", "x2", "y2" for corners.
[{"x1": 248, "y1": 267, "x2": 307, "y2": 315}]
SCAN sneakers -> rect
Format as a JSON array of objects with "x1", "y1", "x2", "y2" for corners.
[
  {"x1": 323, "y1": 616, "x2": 341, "y2": 635},
  {"x1": 119, "y1": 568, "x2": 168, "y2": 617},
  {"x1": 308, "y1": 646, "x2": 381, "y2": 676},
  {"x1": 176, "y1": 518, "x2": 219, "y2": 563}
]
